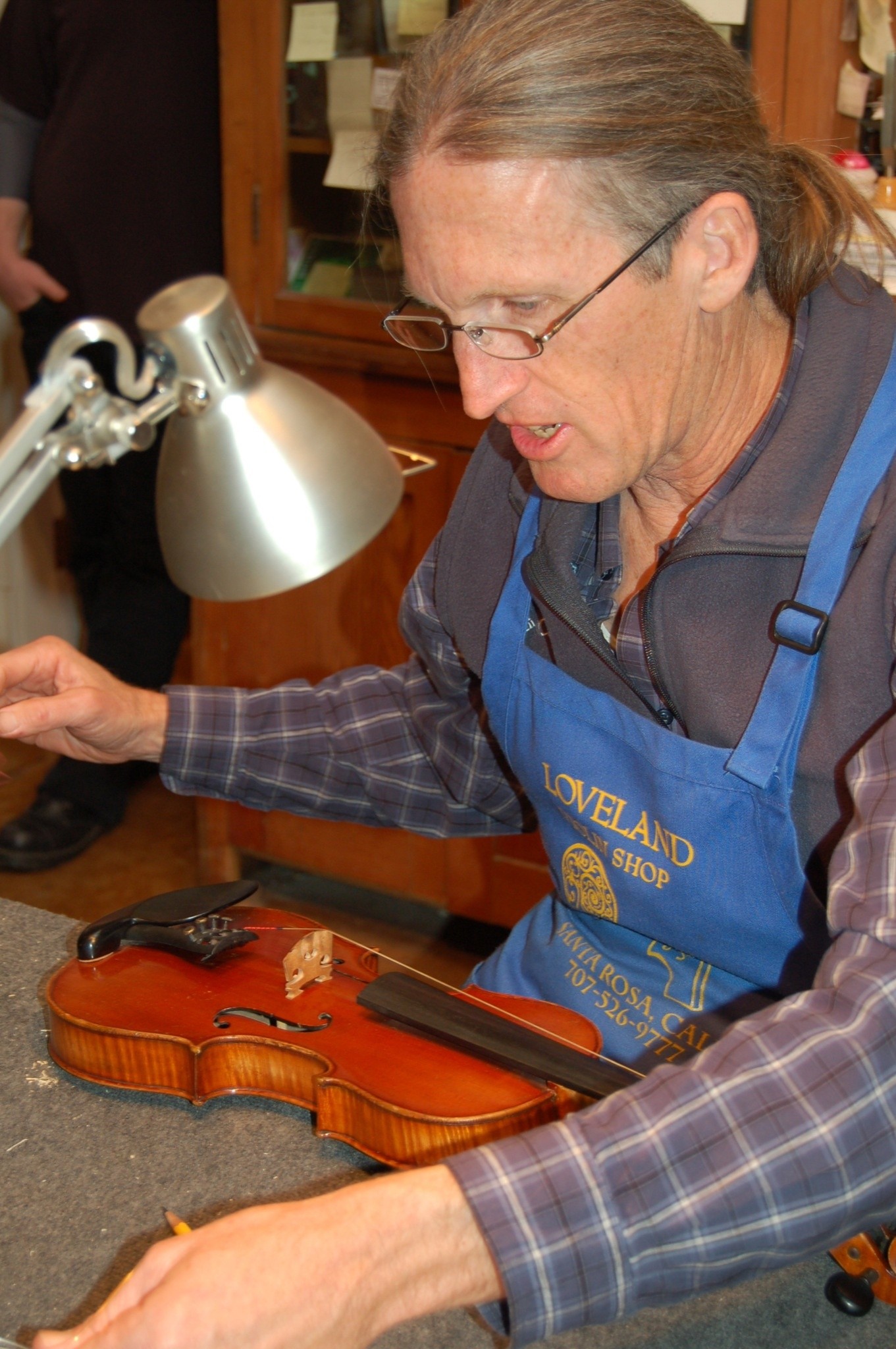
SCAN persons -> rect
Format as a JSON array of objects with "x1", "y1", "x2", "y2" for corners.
[
  {"x1": 0, "y1": 0, "x2": 896, "y2": 1349},
  {"x1": 0, "y1": 0, "x2": 224, "y2": 868}
]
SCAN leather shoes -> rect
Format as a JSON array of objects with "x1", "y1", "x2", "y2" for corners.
[{"x1": 0, "y1": 787, "x2": 123, "y2": 872}]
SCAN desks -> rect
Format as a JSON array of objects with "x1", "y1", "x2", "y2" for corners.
[{"x1": 0, "y1": 895, "x2": 896, "y2": 1348}]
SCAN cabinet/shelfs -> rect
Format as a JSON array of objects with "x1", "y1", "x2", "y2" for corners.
[{"x1": 193, "y1": 0, "x2": 896, "y2": 929}]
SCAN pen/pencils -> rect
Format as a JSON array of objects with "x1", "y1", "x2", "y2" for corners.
[{"x1": 163, "y1": 1209, "x2": 192, "y2": 1236}]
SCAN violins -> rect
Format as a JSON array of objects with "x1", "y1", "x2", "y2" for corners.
[{"x1": 44, "y1": 879, "x2": 896, "y2": 1317}]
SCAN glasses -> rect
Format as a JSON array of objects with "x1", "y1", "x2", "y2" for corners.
[{"x1": 381, "y1": 199, "x2": 703, "y2": 360}]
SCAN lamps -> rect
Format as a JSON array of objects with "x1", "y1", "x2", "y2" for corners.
[{"x1": 1, "y1": 275, "x2": 437, "y2": 601}]
